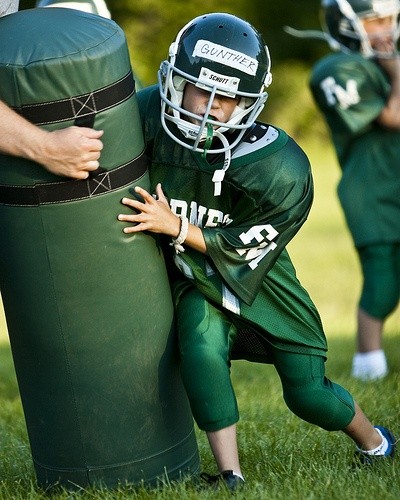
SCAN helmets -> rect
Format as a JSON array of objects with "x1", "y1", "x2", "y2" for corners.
[
  {"x1": 323, "y1": 0, "x2": 400, "y2": 58},
  {"x1": 156, "y1": 12, "x2": 272, "y2": 153}
]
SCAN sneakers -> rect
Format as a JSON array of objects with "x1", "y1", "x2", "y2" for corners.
[
  {"x1": 353, "y1": 424, "x2": 396, "y2": 475},
  {"x1": 211, "y1": 470, "x2": 247, "y2": 498},
  {"x1": 350, "y1": 350, "x2": 389, "y2": 383}
]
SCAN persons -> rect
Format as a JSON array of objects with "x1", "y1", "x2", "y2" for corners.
[
  {"x1": 0, "y1": 0, "x2": 105, "y2": 181},
  {"x1": 301, "y1": 1, "x2": 400, "y2": 383},
  {"x1": 113, "y1": 12, "x2": 400, "y2": 492}
]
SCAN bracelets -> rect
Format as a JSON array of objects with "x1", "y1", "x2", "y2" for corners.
[{"x1": 170, "y1": 214, "x2": 190, "y2": 255}]
